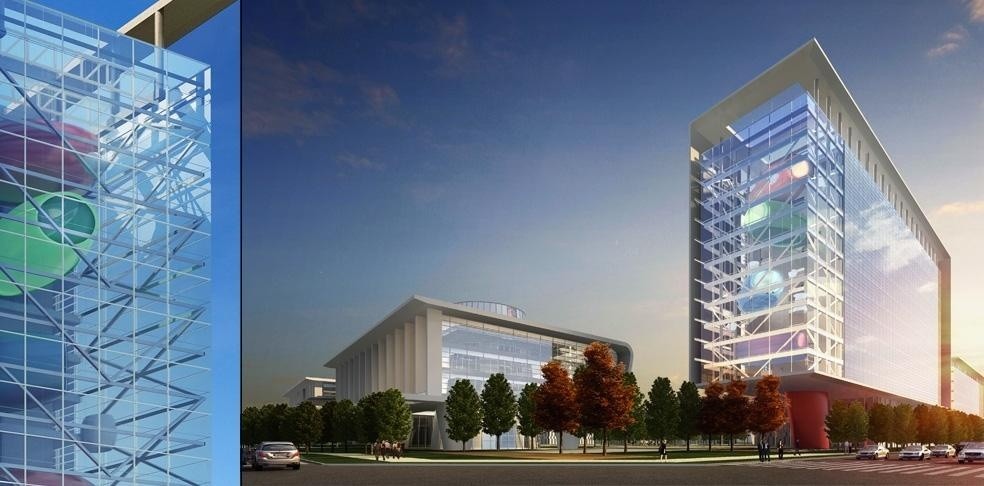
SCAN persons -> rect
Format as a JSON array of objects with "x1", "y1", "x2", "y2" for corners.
[
  {"x1": 757, "y1": 439, "x2": 765, "y2": 462},
  {"x1": 793, "y1": 438, "x2": 801, "y2": 456},
  {"x1": 763, "y1": 439, "x2": 770, "y2": 461},
  {"x1": 372, "y1": 439, "x2": 405, "y2": 461},
  {"x1": 844, "y1": 440, "x2": 850, "y2": 456},
  {"x1": 659, "y1": 440, "x2": 667, "y2": 461},
  {"x1": 849, "y1": 440, "x2": 853, "y2": 453},
  {"x1": 776, "y1": 439, "x2": 783, "y2": 460}
]
